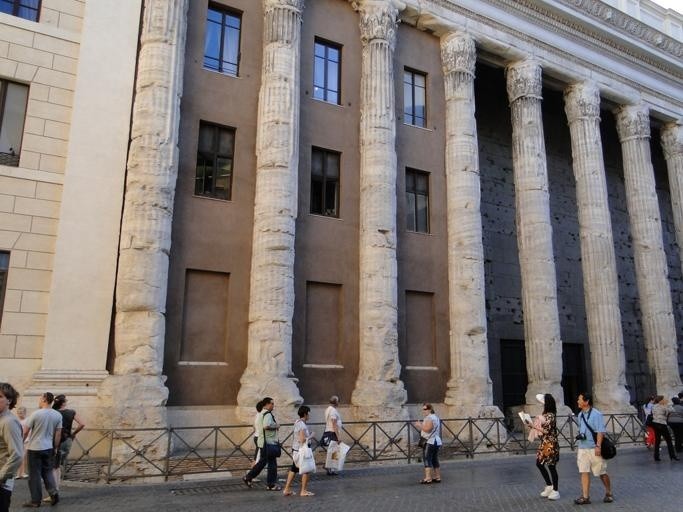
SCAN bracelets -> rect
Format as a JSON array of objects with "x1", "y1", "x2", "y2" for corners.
[{"x1": 596, "y1": 445, "x2": 601, "y2": 448}]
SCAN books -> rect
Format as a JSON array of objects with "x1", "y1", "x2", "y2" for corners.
[{"x1": 518, "y1": 410, "x2": 533, "y2": 425}]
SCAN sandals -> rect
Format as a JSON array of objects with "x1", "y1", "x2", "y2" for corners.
[
  {"x1": 266, "y1": 484, "x2": 281, "y2": 491},
  {"x1": 283, "y1": 491, "x2": 295, "y2": 496},
  {"x1": 419, "y1": 480, "x2": 431, "y2": 484},
  {"x1": 300, "y1": 492, "x2": 314, "y2": 496},
  {"x1": 432, "y1": 478, "x2": 441, "y2": 482},
  {"x1": 242, "y1": 476, "x2": 253, "y2": 488},
  {"x1": 604, "y1": 495, "x2": 612, "y2": 502},
  {"x1": 574, "y1": 497, "x2": 590, "y2": 505}
]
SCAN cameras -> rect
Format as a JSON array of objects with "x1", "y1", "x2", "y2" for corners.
[{"x1": 575, "y1": 433, "x2": 587, "y2": 440}]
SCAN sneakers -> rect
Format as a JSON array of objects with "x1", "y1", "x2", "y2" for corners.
[
  {"x1": 547, "y1": 490, "x2": 559, "y2": 500},
  {"x1": 540, "y1": 485, "x2": 552, "y2": 497}
]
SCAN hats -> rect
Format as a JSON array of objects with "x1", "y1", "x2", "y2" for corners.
[{"x1": 535, "y1": 394, "x2": 545, "y2": 405}]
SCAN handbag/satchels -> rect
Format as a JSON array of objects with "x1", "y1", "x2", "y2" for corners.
[
  {"x1": 264, "y1": 444, "x2": 280, "y2": 457},
  {"x1": 591, "y1": 431, "x2": 615, "y2": 460},
  {"x1": 319, "y1": 432, "x2": 337, "y2": 446},
  {"x1": 417, "y1": 437, "x2": 426, "y2": 449},
  {"x1": 645, "y1": 414, "x2": 652, "y2": 425}
]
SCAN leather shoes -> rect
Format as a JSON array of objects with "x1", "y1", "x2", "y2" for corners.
[
  {"x1": 327, "y1": 470, "x2": 339, "y2": 476},
  {"x1": 669, "y1": 456, "x2": 679, "y2": 461},
  {"x1": 22, "y1": 493, "x2": 61, "y2": 507}
]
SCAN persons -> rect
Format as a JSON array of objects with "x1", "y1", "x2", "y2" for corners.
[
  {"x1": 640, "y1": 391, "x2": 683, "y2": 462},
  {"x1": 525, "y1": 392, "x2": 560, "y2": 500},
  {"x1": 282, "y1": 404, "x2": 317, "y2": 496},
  {"x1": 573, "y1": 391, "x2": 614, "y2": 505},
  {"x1": 319, "y1": 395, "x2": 342, "y2": 476},
  {"x1": 0, "y1": 381, "x2": 84, "y2": 512},
  {"x1": 251, "y1": 400, "x2": 264, "y2": 482},
  {"x1": 415, "y1": 403, "x2": 443, "y2": 483},
  {"x1": 241, "y1": 396, "x2": 282, "y2": 491}
]
